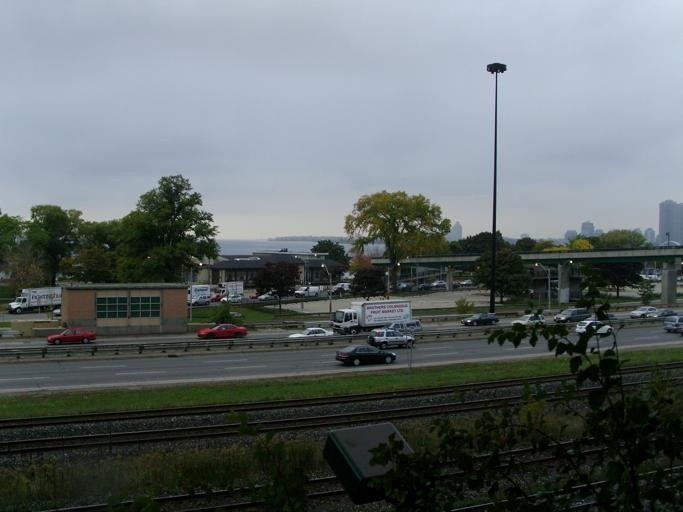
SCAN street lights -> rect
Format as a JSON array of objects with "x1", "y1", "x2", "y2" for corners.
[
  {"x1": 557, "y1": 260, "x2": 573, "y2": 303},
  {"x1": 319, "y1": 263, "x2": 334, "y2": 313},
  {"x1": 294, "y1": 255, "x2": 308, "y2": 285},
  {"x1": 188, "y1": 261, "x2": 204, "y2": 321},
  {"x1": 314, "y1": 254, "x2": 321, "y2": 260},
  {"x1": 484, "y1": 61, "x2": 508, "y2": 314},
  {"x1": 534, "y1": 262, "x2": 552, "y2": 310}
]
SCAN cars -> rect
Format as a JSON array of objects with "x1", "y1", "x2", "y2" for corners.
[
  {"x1": 391, "y1": 277, "x2": 475, "y2": 291},
  {"x1": 553, "y1": 303, "x2": 683, "y2": 337},
  {"x1": 186, "y1": 280, "x2": 353, "y2": 306},
  {"x1": 334, "y1": 344, "x2": 397, "y2": 366},
  {"x1": 52, "y1": 307, "x2": 61, "y2": 318},
  {"x1": 458, "y1": 311, "x2": 501, "y2": 326},
  {"x1": 196, "y1": 322, "x2": 248, "y2": 340},
  {"x1": 47, "y1": 326, "x2": 97, "y2": 346},
  {"x1": 658, "y1": 240, "x2": 680, "y2": 249},
  {"x1": 288, "y1": 326, "x2": 334, "y2": 339}
]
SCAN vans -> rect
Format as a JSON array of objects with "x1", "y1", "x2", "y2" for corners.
[{"x1": 382, "y1": 320, "x2": 424, "y2": 338}]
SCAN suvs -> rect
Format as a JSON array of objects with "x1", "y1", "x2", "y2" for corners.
[
  {"x1": 366, "y1": 326, "x2": 416, "y2": 350},
  {"x1": 513, "y1": 313, "x2": 548, "y2": 328}
]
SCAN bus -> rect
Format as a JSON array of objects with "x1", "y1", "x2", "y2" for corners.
[{"x1": 638, "y1": 267, "x2": 657, "y2": 281}]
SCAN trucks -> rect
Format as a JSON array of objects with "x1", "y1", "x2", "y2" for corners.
[
  {"x1": 7, "y1": 286, "x2": 63, "y2": 315},
  {"x1": 332, "y1": 296, "x2": 413, "y2": 336}
]
